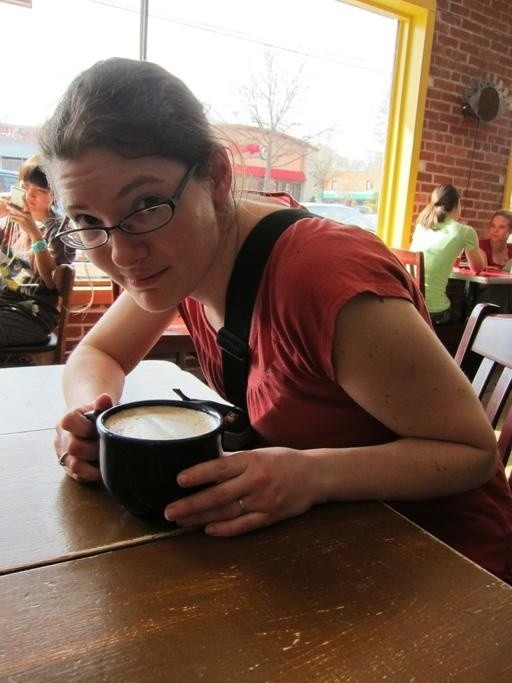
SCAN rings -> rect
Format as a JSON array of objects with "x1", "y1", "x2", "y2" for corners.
[
  {"x1": 238, "y1": 498, "x2": 246, "y2": 518},
  {"x1": 59, "y1": 450, "x2": 70, "y2": 465}
]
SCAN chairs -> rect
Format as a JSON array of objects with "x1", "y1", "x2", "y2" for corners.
[
  {"x1": 385, "y1": 245, "x2": 426, "y2": 305},
  {"x1": 454, "y1": 302, "x2": 512, "y2": 484},
  {"x1": 0, "y1": 261, "x2": 75, "y2": 365}
]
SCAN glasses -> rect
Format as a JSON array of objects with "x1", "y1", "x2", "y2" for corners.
[{"x1": 54, "y1": 163, "x2": 197, "y2": 248}]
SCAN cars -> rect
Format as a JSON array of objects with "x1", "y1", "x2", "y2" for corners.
[
  {"x1": 299, "y1": 201, "x2": 378, "y2": 234},
  {"x1": 0, "y1": 167, "x2": 74, "y2": 231}
]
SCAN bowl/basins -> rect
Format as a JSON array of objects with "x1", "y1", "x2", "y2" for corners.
[{"x1": 185, "y1": 397, "x2": 254, "y2": 451}]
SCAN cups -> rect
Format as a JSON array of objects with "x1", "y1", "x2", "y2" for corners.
[{"x1": 81, "y1": 398, "x2": 226, "y2": 526}]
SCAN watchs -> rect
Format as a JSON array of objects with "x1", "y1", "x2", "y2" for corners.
[{"x1": 31, "y1": 241, "x2": 48, "y2": 252}]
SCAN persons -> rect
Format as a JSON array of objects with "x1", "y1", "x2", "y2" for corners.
[
  {"x1": 34, "y1": 57, "x2": 511, "y2": 590},
  {"x1": 0, "y1": 151, "x2": 72, "y2": 348},
  {"x1": 476, "y1": 207, "x2": 512, "y2": 274},
  {"x1": 409, "y1": 181, "x2": 487, "y2": 327}
]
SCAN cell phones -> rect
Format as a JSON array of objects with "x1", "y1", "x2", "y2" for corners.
[{"x1": 10, "y1": 185, "x2": 27, "y2": 211}]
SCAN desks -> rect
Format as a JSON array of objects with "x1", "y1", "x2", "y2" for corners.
[
  {"x1": 1, "y1": 359, "x2": 237, "y2": 572},
  {"x1": 451, "y1": 264, "x2": 512, "y2": 313},
  {"x1": 0, "y1": 502, "x2": 511, "y2": 682}
]
